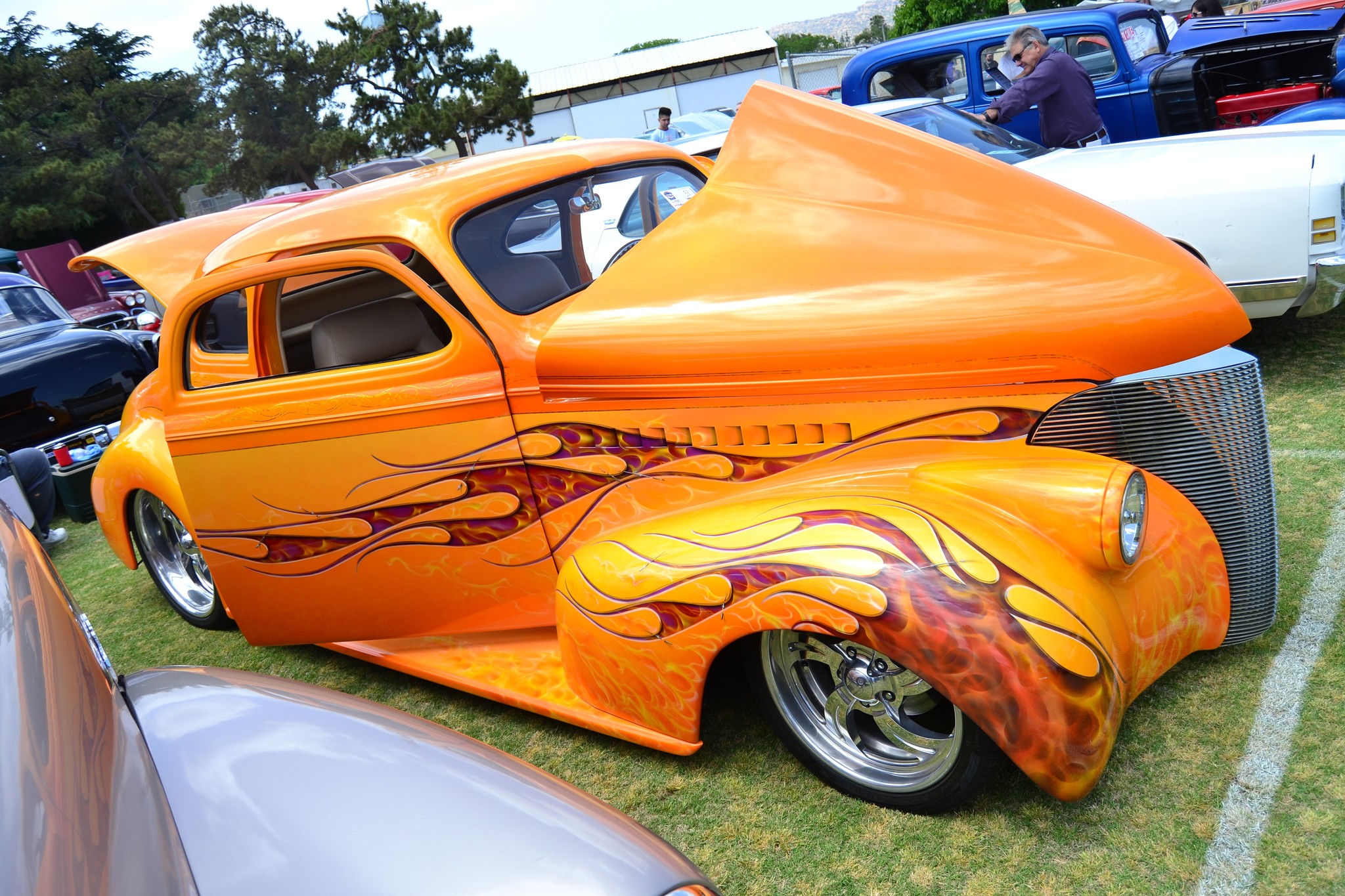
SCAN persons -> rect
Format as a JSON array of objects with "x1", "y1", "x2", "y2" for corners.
[
  {"x1": 957, "y1": 25, "x2": 1112, "y2": 150},
  {"x1": 984, "y1": 53, "x2": 999, "y2": 71},
  {"x1": 580, "y1": 196, "x2": 593, "y2": 213},
  {"x1": 996, "y1": 49, "x2": 1035, "y2": 90},
  {"x1": 1191, "y1": 0, "x2": 1225, "y2": 17},
  {"x1": 970, "y1": 129, "x2": 1005, "y2": 148},
  {"x1": 652, "y1": 107, "x2": 680, "y2": 143},
  {"x1": 1112, "y1": 0, "x2": 1178, "y2": 56},
  {"x1": 0, "y1": 448, "x2": 69, "y2": 546}
]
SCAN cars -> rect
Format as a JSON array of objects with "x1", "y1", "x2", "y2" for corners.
[
  {"x1": 0, "y1": 2, "x2": 1344, "y2": 466},
  {"x1": 90, "y1": 77, "x2": 1286, "y2": 807}
]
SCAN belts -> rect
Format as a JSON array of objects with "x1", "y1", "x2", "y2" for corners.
[{"x1": 1065, "y1": 129, "x2": 1107, "y2": 149}]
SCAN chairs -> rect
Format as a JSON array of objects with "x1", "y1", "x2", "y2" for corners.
[
  {"x1": 309, "y1": 295, "x2": 442, "y2": 367},
  {"x1": 475, "y1": 255, "x2": 570, "y2": 309}
]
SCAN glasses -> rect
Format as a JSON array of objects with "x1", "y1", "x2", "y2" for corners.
[
  {"x1": 989, "y1": 55, "x2": 992, "y2": 57},
  {"x1": 1192, "y1": 12, "x2": 1202, "y2": 18},
  {"x1": 1012, "y1": 41, "x2": 1040, "y2": 63}
]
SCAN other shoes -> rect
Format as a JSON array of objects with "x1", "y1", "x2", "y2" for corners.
[{"x1": 42, "y1": 527, "x2": 67, "y2": 549}]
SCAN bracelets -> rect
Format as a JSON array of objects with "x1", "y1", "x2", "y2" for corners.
[{"x1": 980, "y1": 110, "x2": 992, "y2": 124}]
